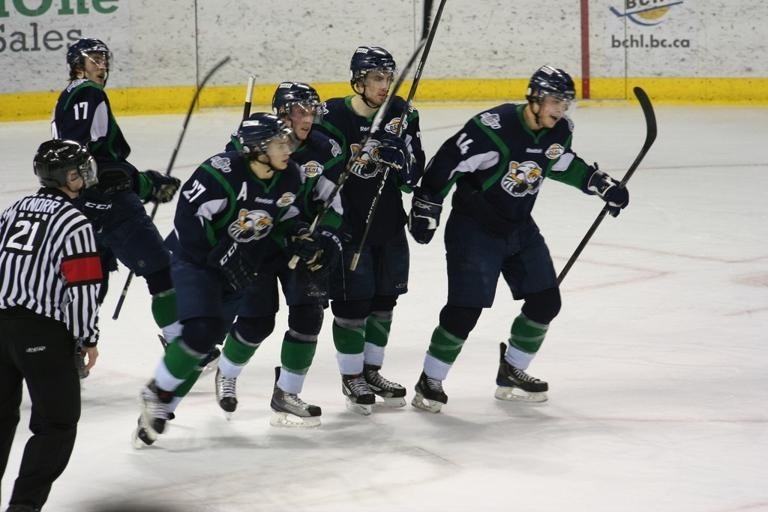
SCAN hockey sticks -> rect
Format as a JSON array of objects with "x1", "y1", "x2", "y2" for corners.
[
  {"x1": 288, "y1": 0, "x2": 447, "y2": 272},
  {"x1": 348, "y1": 2, "x2": 447, "y2": 273},
  {"x1": 555, "y1": 87, "x2": 657, "y2": 284},
  {"x1": 288, "y1": 1, "x2": 434, "y2": 270},
  {"x1": 113, "y1": 53, "x2": 231, "y2": 320}
]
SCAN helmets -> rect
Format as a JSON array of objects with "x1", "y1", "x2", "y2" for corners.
[
  {"x1": 237, "y1": 113, "x2": 291, "y2": 159},
  {"x1": 349, "y1": 47, "x2": 396, "y2": 93},
  {"x1": 32, "y1": 140, "x2": 98, "y2": 198},
  {"x1": 271, "y1": 82, "x2": 323, "y2": 124},
  {"x1": 525, "y1": 66, "x2": 575, "y2": 118},
  {"x1": 66, "y1": 38, "x2": 113, "y2": 81}
]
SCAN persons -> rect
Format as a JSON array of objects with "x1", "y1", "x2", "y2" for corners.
[
  {"x1": 310, "y1": 46, "x2": 425, "y2": 405},
  {"x1": 137, "y1": 112, "x2": 322, "y2": 448},
  {"x1": 0, "y1": 138, "x2": 103, "y2": 511},
  {"x1": 216, "y1": 82, "x2": 346, "y2": 418},
  {"x1": 51, "y1": 39, "x2": 221, "y2": 379},
  {"x1": 408, "y1": 64, "x2": 630, "y2": 404}
]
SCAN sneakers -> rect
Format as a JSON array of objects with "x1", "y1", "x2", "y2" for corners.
[
  {"x1": 215, "y1": 368, "x2": 236, "y2": 411},
  {"x1": 138, "y1": 414, "x2": 158, "y2": 444},
  {"x1": 76, "y1": 350, "x2": 88, "y2": 379},
  {"x1": 364, "y1": 364, "x2": 406, "y2": 398},
  {"x1": 415, "y1": 371, "x2": 448, "y2": 404},
  {"x1": 496, "y1": 362, "x2": 548, "y2": 392},
  {"x1": 342, "y1": 374, "x2": 375, "y2": 404},
  {"x1": 142, "y1": 381, "x2": 164, "y2": 433},
  {"x1": 271, "y1": 383, "x2": 321, "y2": 416}
]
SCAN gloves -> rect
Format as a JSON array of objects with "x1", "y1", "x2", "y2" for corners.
[
  {"x1": 77, "y1": 196, "x2": 113, "y2": 234},
  {"x1": 407, "y1": 186, "x2": 443, "y2": 244},
  {"x1": 376, "y1": 133, "x2": 412, "y2": 187},
  {"x1": 581, "y1": 162, "x2": 628, "y2": 218},
  {"x1": 285, "y1": 221, "x2": 344, "y2": 279},
  {"x1": 205, "y1": 241, "x2": 255, "y2": 292},
  {"x1": 142, "y1": 170, "x2": 180, "y2": 203}
]
